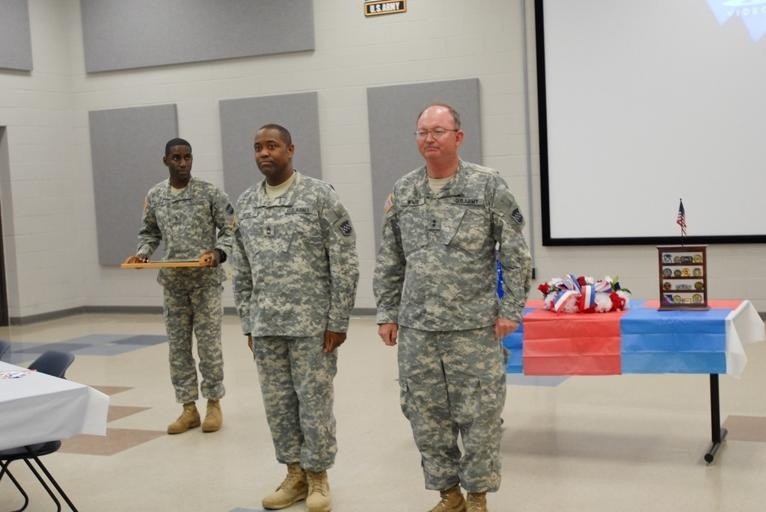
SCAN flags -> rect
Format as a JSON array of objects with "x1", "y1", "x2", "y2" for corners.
[{"x1": 677, "y1": 202, "x2": 686, "y2": 235}]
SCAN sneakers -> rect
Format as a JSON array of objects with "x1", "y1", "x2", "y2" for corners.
[{"x1": 465, "y1": 490, "x2": 488, "y2": 512}]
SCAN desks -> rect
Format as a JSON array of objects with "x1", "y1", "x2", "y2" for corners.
[
  {"x1": 499, "y1": 297, "x2": 766, "y2": 466},
  {"x1": 0, "y1": 359, "x2": 113, "y2": 511}
]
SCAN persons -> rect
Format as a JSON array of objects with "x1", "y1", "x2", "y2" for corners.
[
  {"x1": 228, "y1": 124, "x2": 360, "y2": 509},
  {"x1": 125, "y1": 139, "x2": 235, "y2": 434},
  {"x1": 372, "y1": 103, "x2": 531, "y2": 512}
]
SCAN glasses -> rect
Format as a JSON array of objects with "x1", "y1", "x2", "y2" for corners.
[{"x1": 416, "y1": 126, "x2": 459, "y2": 138}]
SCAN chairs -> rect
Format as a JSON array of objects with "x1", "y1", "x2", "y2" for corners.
[
  {"x1": 0, "y1": 341, "x2": 12, "y2": 362},
  {"x1": 0, "y1": 349, "x2": 79, "y2": 511}
]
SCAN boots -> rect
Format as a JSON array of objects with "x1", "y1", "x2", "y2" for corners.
[
  {"x1": 262, "y1": 462, "x2": 308, "y2": 510},
  {"x1": 166, "y1": 401, "x2": 201, "y2": 435},
  {"x1": 305, "y1": 468, "x2": 334, "y2": 512},
  {"x1": 201, "y1": 398, "x2": 224, "y2": 433},
  {"x1": 428, "y1": 483, "x2": 467, "y2": 512}
]
